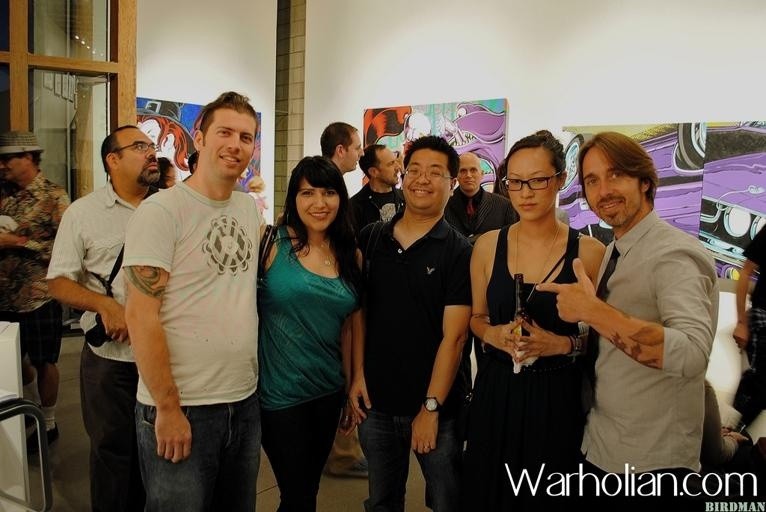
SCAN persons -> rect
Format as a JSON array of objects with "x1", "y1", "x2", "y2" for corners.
[
  {"x1": 0, "y1": 132, "x2": 74, "y2": 456},
  {"x1": 350, "y1": 134, "x2": 474, "y2": 512},
  {"x1": 132, "y1": 99, "x2": 198, "y2": 184},
  {"x1": 44, "y1": 124, "x2": 169, "y2": 512},
  {"x1": 464, "y1": 130, "x2": 607, "y2": 512},
  {"x1": 122, "y1": 91, "x2": 262, "y2": 511},
  {"x1": 703, "y1": 377, "x2": 753, "y2": 474},
  {"x1": 732, "y1": 224, "x2": 766, "y2": 433},
  {"x1": 536, "y1": 131, "x2": 721, "y2": 511},
  {"x1": 444, "y1": 151, "x2": 519, "y2": 244},
  {"x1": 320, "y1": 122, "x2": 364, "y2": 174},
  {"x1": 348, "y1": 145, "x2": 406, "y2": 235},
  {"x1": 152, "y1": 153, "x2": 179, "y2": 193},
  {"x1": 255, "y1": 155, "x2": 357, "y2": 512},
  {"x1": 187, "y1": 149, "x2": 200, "y2": 177}
]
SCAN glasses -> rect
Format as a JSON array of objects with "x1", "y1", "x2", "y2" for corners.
[
  {"x1": 116, "y1": 141, "x2": 157, "y2": 153},
  {"x1": 402, "y1": 166, "x2": 452, "y2": 182},
  {"x1": 503, "y1": 173, "x2": 561, "y2": 191}
]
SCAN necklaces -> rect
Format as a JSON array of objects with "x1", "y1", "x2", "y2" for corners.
[
  {"x1": 314, "y1": 244, "x2": 335, "y2": 269},
  {"x1": 513, "y1": 226, "x2": 564, "y2": 303}
]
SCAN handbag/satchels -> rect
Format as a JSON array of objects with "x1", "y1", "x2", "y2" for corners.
[{"x1": 326, "y1": 421, "x2": 368, "y2": 482}]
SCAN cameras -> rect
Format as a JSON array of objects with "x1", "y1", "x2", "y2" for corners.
[{"x1": 84, "y1": 314, "x2": 113, "y2": 348}]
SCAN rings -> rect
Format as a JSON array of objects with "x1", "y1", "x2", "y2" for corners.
[
  {"x1": 423, "y1": 446, "x2": 431, "y2": 449},
  {"x1": 342, "y1": 415, "x2": 352, "y2": 421}
]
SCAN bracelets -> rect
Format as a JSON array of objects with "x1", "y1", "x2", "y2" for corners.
[{"x1": 567, "y1": 334, "x2": 582, "y2": 358}]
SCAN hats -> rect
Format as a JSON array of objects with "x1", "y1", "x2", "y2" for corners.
[{"x1": 1, "y1": 131, "x2": 44, "y2": 156}]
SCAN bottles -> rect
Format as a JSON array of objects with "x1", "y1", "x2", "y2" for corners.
[{"x1": 509, "y1": 273, "x2": 532, "y2": 338}]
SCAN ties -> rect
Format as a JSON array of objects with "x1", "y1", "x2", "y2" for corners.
[
  {"x1": 461, "y1": 199, "x2": 477, "y2": 225},
  {"x1": 583, "y1": 244, "x2": 621, "y2": 409}
]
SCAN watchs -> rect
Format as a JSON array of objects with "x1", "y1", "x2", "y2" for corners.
[{"x1": 422, "y1": 397, "x2": 442, "y2": 413}]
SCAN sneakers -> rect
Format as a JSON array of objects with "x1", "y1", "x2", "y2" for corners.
[{"x1": 42, "y1": 422, "x2": 59, "y2": 453}]
